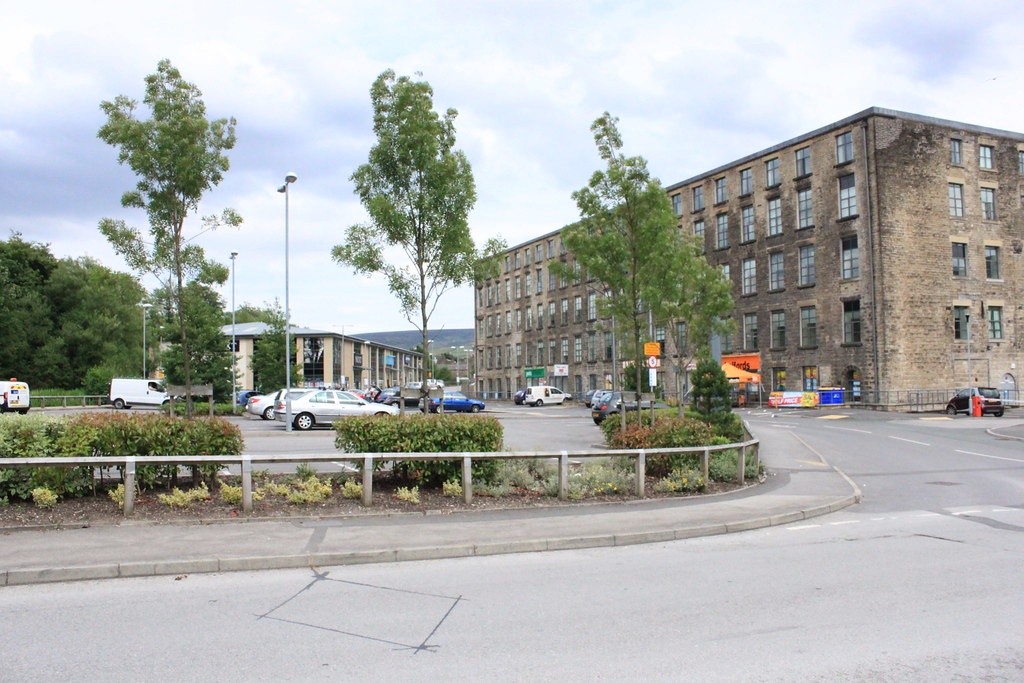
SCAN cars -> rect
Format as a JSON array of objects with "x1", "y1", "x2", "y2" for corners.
[
  {"x1": 274, "y1": 387, "x2": 319, "y2": 400},
  {"x1": 273, "y1": 389, "x2": 400, "y2": 430},
  {"x1": 427, "y1": 379, "x2": 445, "y2": 390},
  {"x1": 585, "y1": 389, "x2": 671, "y2": 425},
  {"x1": 348, "y1": 382, "x2": 422, "y2": 409},
  {"x1": 514, "y1": 390, "x2": 526, "y2": 405},
  {"x1": 945, "y1": 387, "x2": 1004, "y2": 417},
  {"x1": 236, "y1": 391, "x2": 264, "y2": 406},
  {"x1": 246, "y1": 392, "x2": 279, "y2": 420},
  {"x1": 418, "y1": 391, "x2": 485, "y2": 414}
]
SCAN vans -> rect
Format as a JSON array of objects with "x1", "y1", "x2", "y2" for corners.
[
  {"x1": 0, "y1": 378, "x2": 31, "y2": 415},
  {"x1": 110, "y1": 378, "x2": 178, "y2": 410},
  {"x1": 525, "y1": 386, "x2": 572, "y2": 407}
]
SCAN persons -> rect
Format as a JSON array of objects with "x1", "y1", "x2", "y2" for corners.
[
  {"x1": 148, "y1": 383, "x2": 159, "y2": 391},
  {"x1": 318, "y1": 386, "x2": 345, "y2": 391},
  {"x1": 365, "y1": 386, "x2": 372, "y2": 403}
]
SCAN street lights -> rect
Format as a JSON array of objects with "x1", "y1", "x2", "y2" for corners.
[
  {"x1": 428, "y1": 340, "x2": 434, "y2": 379},
  {"x1": 450, "y1": 346, "x2": 464, "y2": 386},
  {"x1": 229, "y1": 252, "x2": 238, "y2": 413},
  {"x1": 276, "y1": 172, "x2": 298, "y2": 431},
  {"x1": 364, "y1": 341, "x2": 370, "y2": 388},
  {"x1": 464, "y1": 349, "x2": 473, "y2": 384},
  {"x1": 135, "y1": 303, "x2": 153, "y2": 379}
]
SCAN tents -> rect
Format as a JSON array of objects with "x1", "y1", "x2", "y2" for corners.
[{"x1": 721, "y1": 362, "x2": 762, "y2": 409}]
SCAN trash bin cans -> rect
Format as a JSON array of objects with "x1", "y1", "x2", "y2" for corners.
[{"x1": 972, "y1": 396, "x2": 982, "y2": 416}]
